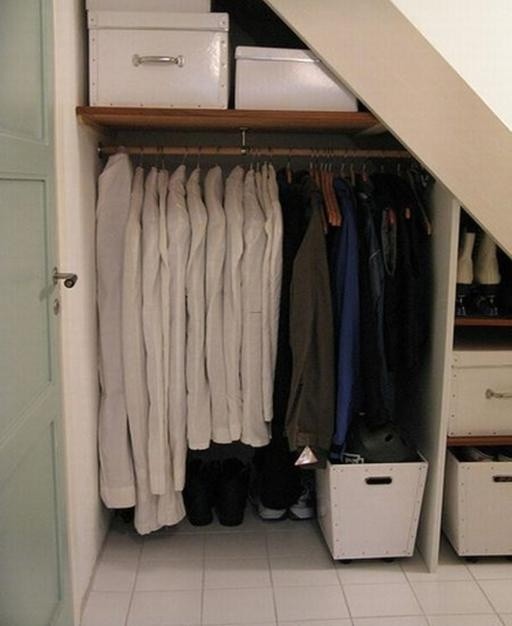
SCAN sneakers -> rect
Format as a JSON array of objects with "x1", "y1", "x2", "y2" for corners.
[
  {"x1": 248, "y1": 485, "x2": 291, "y2": 520},
  {"x1": 289, "y1": 470, "x2": 316, "y2": 520}
]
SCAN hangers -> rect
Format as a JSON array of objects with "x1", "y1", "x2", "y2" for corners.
[{"x1": 96, "y1": 145, "x2": 429, "y2": 227}]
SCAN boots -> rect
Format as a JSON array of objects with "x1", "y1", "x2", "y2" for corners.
[
  {"x1": 214, "y1": 458, "x2": 246, "y2": 527},
  {"x1": 184, "y1": 458, "x2": 214, "y2": 526}
]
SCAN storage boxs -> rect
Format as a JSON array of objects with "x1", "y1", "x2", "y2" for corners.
[
  {"x1": 235, "y1": 46, "x2": 357, "y2": 111},
  {"x1": 450, "y1": 351, "x2": 511, "y2": 435},
  {"x1": 447, "y1": 449, "x2": 512, "y2": 556},
  {"x1": 92, "y1": 12, "x2": 228, "y2": 108},
  {"x1": 315, "y1": 441, "x2": 427, "y2": 560}
]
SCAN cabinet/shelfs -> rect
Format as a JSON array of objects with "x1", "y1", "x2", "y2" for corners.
[{"x1": 431, "y1": 200, "x2": 512, "y2": 568}]
